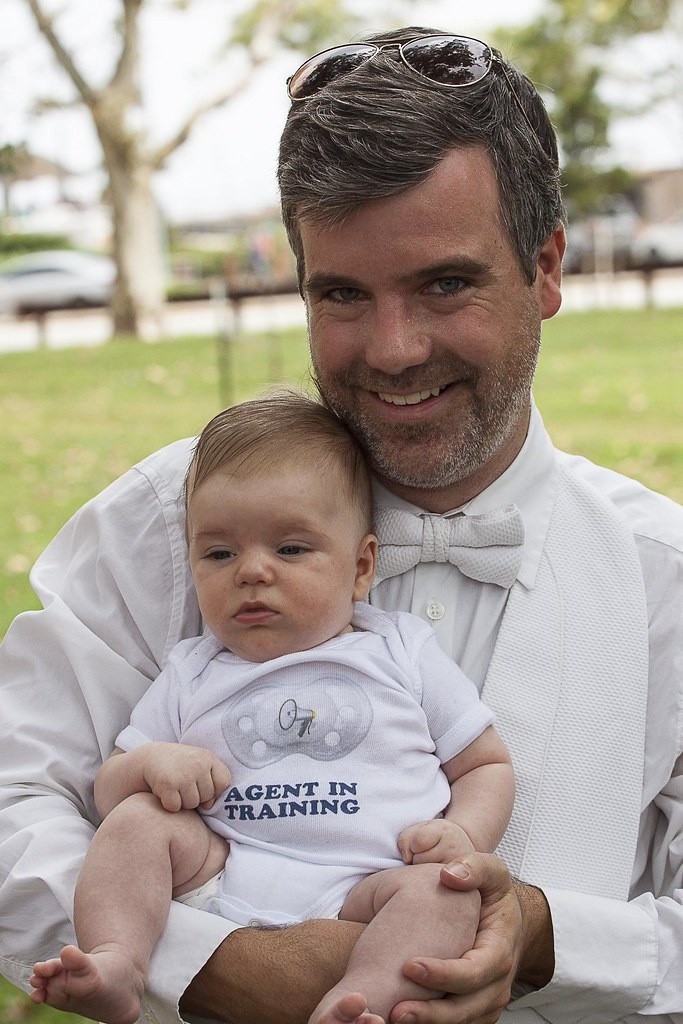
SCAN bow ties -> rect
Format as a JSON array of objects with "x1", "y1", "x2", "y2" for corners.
[{"x1": 371, "y1": 502, "x2": 525, "y2": 589}]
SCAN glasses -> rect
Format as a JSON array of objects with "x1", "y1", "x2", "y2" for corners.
[{"x1": 279, "y1": 33, "x2": 540, "y2": 145}]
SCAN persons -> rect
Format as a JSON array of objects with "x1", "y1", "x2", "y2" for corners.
[
  {"x1": 0, "y1": 29, "x2": 683, "y2": 1024},
  {"x1": 27, "y1": 398, "x2": 516, "y2": 1024}
]
